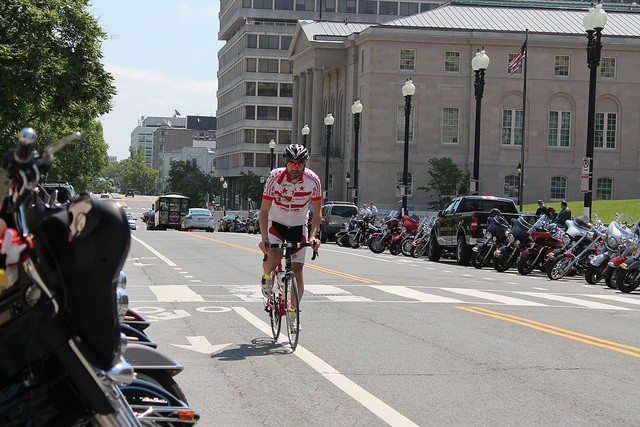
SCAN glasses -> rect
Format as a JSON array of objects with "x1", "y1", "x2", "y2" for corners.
[{"x1": 285, "y1": 161, "x2": 306, "y2": 168}]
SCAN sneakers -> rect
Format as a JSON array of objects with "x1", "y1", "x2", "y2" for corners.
[
  {"x1": 261, "y1": 278, "x2": 272, "y2": 298},
  {"x1": 290, "y1": 318, "x2": 301, "y2": 334}
]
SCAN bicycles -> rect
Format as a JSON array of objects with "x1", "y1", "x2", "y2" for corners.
[{"x1": 258, "y1": 238, "x2": 321, "y2": 349}]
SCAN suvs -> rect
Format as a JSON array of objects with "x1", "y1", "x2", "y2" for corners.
[
  {"x1": 125, "y1": 188, "x2": 134, "y2": 197},
  {"x1": 316, "y1": 199, "x2": 360, "y2": 244}
]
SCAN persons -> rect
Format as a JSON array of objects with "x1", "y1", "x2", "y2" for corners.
[
  {"x1": 546, "y1": 206, "x2": 558, "y2": 218},
  {"x1": 360, "y1": 203, "x2": 372, "y2": 217},
  {"x1": 396, "y1": 199, "x2": 403, "y2": 218},
  {"x1": 367, "y1": 201, "x2": 378, "y2": 215},
  {"x1": 535, "y1": 199, "x2": 546, "y2": 215},
  {"x1": 259, "y1": 143, "x2": 323, "y2": 334},
  {"x1": 554, "y1": 201, "x2": 571, "y2": 227}
]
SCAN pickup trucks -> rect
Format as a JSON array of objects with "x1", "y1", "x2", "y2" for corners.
[{"x1": 428, "y1": 195, "x2": 524, "y2": 265}]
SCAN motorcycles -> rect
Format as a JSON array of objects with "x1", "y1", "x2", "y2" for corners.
[
  {"x1": 617, "y1": 219, "x2": 640, "y2": 293},
  {"x1": 470, "y1": 207, "x2": 514, "y2": 269},
  {"x1": 584, "y1": 211, "x2": 635, "y2": 285},
  {"x1": 368, "y1": 208, "x2": 401, "y2": 253},
  {"x1": 604, "y1": 212, "x2": 636, "y2": 288},
  {"x1": 399, "y1": 214, "x2": 428, "y2": 256},
  {"x1": 228, "y1": 215, "x2": 246, "y2": 233},
  {"x1": 334, "y1": 213, "x2": 364, "y2": 246},
  {"x1": 545, "y1": 211, "x2": 609, "y2": 280},
  {"x1": 409, "y1": 215, "x2": 437, "y2": 258},
  {"x1": 346, "y1": 217, "x2": 385, "y2": 248},
  {"x1": 515, "y1": 211, "x2": 566, "y2": 275},
  {"x1": 492, "y1": 210, "x2": 535, "y2": 273},
  {"x1": 389, "y1": 214, "x2": 422, "y2": 255},
  {"x1": 3, "y1": 127, "x2": 160, "y2": 348},
  {"x1": 1, "y1": 123, "x2": 201, "y2": 426}
]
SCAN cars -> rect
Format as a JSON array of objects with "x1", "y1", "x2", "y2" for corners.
[
  {"x1": 180, "y1": 206, "x2": 215, "y2": 232},
  {"x1": 125, "y1": 215, "x2": 137, "y2": 229},
  {"x1": 100, "y1": 191, "x2": 109, "y2": 198},
  {"x1": 147, "y1": 211, "x2": 167, "y2": 230},
  {"x1": 246, "y1": 209, "x2": 261, "y2": 233},
  {"x1": 216, "y1": 213, "x2": 245, "y2": 231}
]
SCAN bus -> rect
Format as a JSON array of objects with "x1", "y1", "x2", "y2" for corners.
[{"x1": 154, "y1": 191, "x2": 191, "y2": 229}]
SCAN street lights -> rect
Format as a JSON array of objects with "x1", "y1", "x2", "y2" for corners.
[
  {"x1": 219, "y1": 175, "x2": 228, "y2": 216},
  {"x1": 269, "y1": 139, "x2": 276, "y2": 172},
  {"x1": 324, "y1": 110, "x2": 335, "y2": 205},
  {"x1": 165, "y1": 175, "x2": 168, "y2": 189},
  {"x1": 469, "y1": 45, "x2": 490, "y2": 196},
  {"x1": 398, "y1": 76, "x2": 416, "y2": 216},
  {"x1": 301, "y1": 121, "x2": 311, "y2": 149},
  {"x1": 581, "y1": 2, "x2": 608, "y2": 223},
  {"x1": 345, "y1": 96, "x2": 364, "y2": 206}
]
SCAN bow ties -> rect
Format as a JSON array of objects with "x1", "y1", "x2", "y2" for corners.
[{"x1": 370, "y1": 208, "x2": 372, "y2": 211}]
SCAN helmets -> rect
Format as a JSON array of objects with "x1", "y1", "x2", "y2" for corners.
[
  {"x1": 490, "y1": 208, "x2": 500, "y2": 217},
  {"x1": 283, "y1": 144, "x2": 309, "y2": 161}
]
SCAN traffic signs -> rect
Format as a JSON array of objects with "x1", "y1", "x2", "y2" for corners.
[
  {"x1": 260, "y1": 175, "x2": 265, "y2": 200},
  {"x1": 248, "y1": 195, "x2": 252, "y2": 213},
  {"x1": 515, "y1": 161, "x2": 522, "y2": 205}
]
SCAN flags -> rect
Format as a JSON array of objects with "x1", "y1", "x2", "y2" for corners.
[{"x1": 509, "y1": 40, "x2": 526, "y2": 76}]
